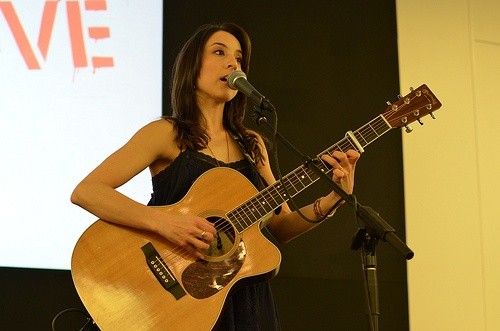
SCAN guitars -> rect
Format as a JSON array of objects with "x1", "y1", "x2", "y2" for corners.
[{"x1": 70, "y1": 83, "x2": 442, "y2": 331}]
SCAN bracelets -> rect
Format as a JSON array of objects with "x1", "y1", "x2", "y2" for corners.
[{"x1": 314, "y1": 197, "x2": 335, "y2": 221}]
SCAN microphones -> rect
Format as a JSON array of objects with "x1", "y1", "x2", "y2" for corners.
[{"x1": 227, "y1": 70, "x2": 276, "y2": 113}]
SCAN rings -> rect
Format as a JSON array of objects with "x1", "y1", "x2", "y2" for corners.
[{"x1": 201, "y1": 231, "x2": 207, "y2": 239}]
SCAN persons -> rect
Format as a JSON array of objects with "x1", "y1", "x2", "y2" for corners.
[{"x1": 70, "y1": 23, "x2": 360, "y2": 331}]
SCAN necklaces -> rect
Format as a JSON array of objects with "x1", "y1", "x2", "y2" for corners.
[{"x1": 207, "y1": 130, "x2": 230, "y2": 168}]
